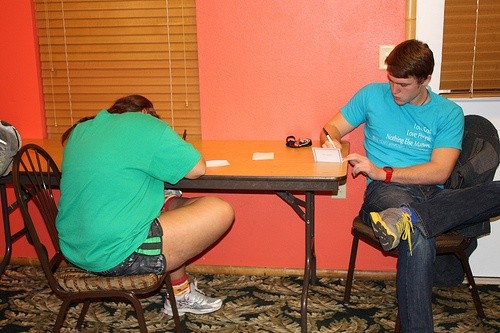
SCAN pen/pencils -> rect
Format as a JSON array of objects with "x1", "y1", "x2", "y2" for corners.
[
  {"x1": 183, "y1": 130, "x2": 186, "y2": 140},
  {"x1": 323, "y1": 128, "x2": 336, "y2": 149}
]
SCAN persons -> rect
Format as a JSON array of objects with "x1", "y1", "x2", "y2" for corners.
[
  {"x1": 55, "y1": 95, "x2": 234, "y2": 315},
  {"x1": 320, "y1": 39, "x2": 500, "y2": 333}
]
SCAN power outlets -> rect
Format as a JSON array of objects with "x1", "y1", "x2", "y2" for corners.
[{"x1": 331, "y1": 184, "x2": 347, "y2": 199}]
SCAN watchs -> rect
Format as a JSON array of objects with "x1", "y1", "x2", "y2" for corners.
[{"x1": 384, "y1": 166, "x2": 394, "y2": 184}]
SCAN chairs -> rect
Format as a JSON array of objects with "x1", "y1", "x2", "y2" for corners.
[
  {"x1": 11, "y1": 143, "x2": 181, "y2": 333},
  {"x1": 341, "y1": 114, "x2": 500, "y2": 320}
]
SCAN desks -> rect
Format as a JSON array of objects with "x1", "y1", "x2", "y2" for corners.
[{"x1": 0, "y1": 137, "x2": 350, "y2": 333}]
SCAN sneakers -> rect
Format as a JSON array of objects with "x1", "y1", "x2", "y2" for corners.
[
  {"x1": 163, "y1": 277, "x2": 222, "y2": 316},
  {"x1": 369, "y1": 207, "x2": 415, "y2": 257}
]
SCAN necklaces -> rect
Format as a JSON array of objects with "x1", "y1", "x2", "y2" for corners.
[{"x1": 419, "y1": 90, "x2": 429, "y2": 106}]
